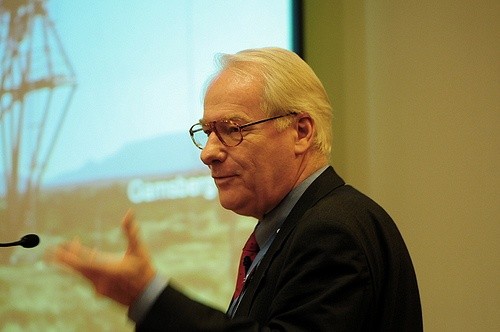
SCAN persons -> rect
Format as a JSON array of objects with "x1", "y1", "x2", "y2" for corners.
[{"x1": 55, "y1": 46, "x2": 423, "y2": 332}]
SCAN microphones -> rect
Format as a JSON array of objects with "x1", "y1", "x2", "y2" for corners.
[{"x1": 0, "y1": 234, "x2": 40, "y2": 248}]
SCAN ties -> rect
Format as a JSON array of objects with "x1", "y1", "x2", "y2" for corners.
[{"x1": 233, "y1": 230, "x2": 261, "y2": 300}]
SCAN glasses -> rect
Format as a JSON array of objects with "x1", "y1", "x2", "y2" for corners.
[{"x1": 190, "y1": 112, "x2": 295, "y2": 150}]
